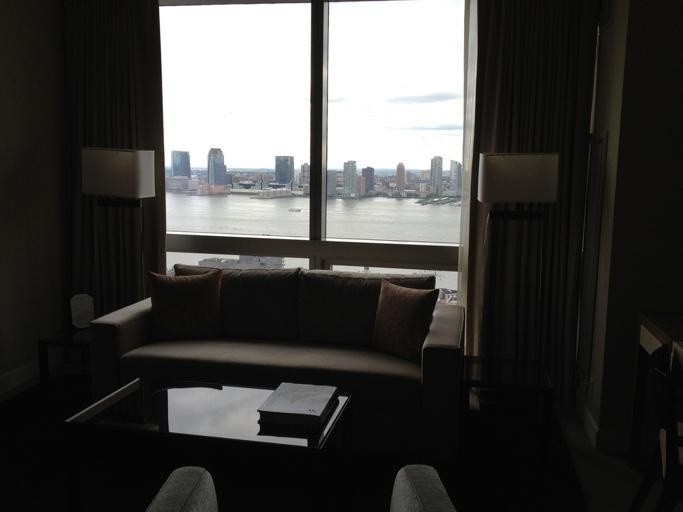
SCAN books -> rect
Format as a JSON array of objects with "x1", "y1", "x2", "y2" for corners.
[{"x1": 257, "y1": 382, "x2": 339, "y2": 427}]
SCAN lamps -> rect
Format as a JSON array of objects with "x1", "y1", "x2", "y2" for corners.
[
  {"x1": 79, "y1": 145, "x2": 156, "y2": 203},
  {"x1": 477, "y1": 152, "x2": 560, "y2": 210}
]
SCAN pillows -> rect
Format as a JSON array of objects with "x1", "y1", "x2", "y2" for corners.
[
  {"x1": 370, "y1": 279, "x2": 439, "y2": 365},
  {"x1": 298, "y1": 269, "x2": 436, "y2": 351},
  {"x1": 144, "y1": 269, "x2": 226, "y2": 341},
  {"x1": 173, "y1": 263, "x2": 300, "y2": 345}
]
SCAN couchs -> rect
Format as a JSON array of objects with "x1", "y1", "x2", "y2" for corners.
[
  {"x1": 90, "y1": 302, "x2": 466, "y2": 441},
  {"x1": 143, "y1": 463, "x2": 456, "y2": 511}
]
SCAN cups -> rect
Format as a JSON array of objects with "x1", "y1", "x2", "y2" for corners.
[{"x1": 71, "y1": 293, "x2": 94, "y2": 329}]
